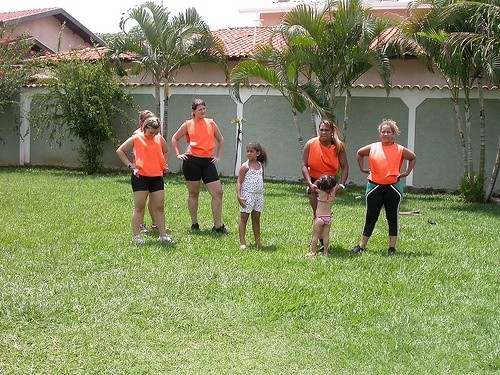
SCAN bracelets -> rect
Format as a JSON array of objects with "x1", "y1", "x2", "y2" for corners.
[
  {"x1": 129, "y1": 162, "x2": 132, "y2": 169},
  {"x1": 339, "y1": 183, "x2": 345, "y2": 189}
]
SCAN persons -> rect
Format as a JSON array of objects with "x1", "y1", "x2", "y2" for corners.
[
  {"x1": 237, "y1": 142, "x2": 268, "y2": 250},
  {"x1": 116, "y1": 109, "x2": 176, "y2": 244},
  {"x1": 172, "y1": 99, "x2": 229, "y2": 235},
  {"x1": 352, "y1": 118, "x2": 417, "y2": 256},
  {"x1": 302, "y1": 121, "x2": 349, "y2": 258}
]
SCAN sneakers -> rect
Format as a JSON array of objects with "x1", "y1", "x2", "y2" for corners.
[
  {"x1": 133, "y1": 235, "x2": 145, "y2": 243},
  {"x1": 351, "y1": 244, "x2": 364, "y2": 254},
  {"x1": 157, "y1": 235, "x2": 174, "y2": 243},
  {"x1": 151, "y1": 224, "x2": 158, "y2": 229},
  {"x1": 139, "y1": 224, "x2": 148, "y2": 232},
  {"x1": 191, "y1": 222, "x2": 200, "y2": 231},
  {"x1": 212, "y1": 224, "x2": 228, "y2": 234},
  {"x1": 388, "y1": 247, "x2": 395, "y2": 256}
]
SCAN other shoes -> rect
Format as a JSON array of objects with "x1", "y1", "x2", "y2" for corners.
[{"x1": 319, "y1": 239, "x2": 324, "y2": 254}]
